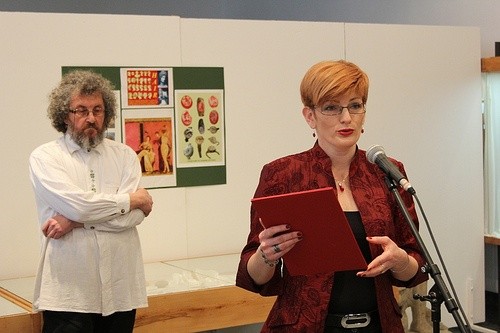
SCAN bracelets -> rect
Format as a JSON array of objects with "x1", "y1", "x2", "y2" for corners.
[
  {"x1": 259, "y1": 245, "x2": 279, "y2": 266},
  {"x1": 391, "y1": 255, "x2": 409, "y2": 273}
]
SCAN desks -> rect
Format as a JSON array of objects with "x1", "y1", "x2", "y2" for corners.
[{"x1": 0, "y1": 252, "x2": 279, "y2": 333}]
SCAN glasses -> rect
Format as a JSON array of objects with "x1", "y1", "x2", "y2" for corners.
[
  {"x1": 67, "y1": 105, "x2": 107, "y2": 118},
  {"x1": 310, "y1": 103, "x2": 366, "y2": 116}
]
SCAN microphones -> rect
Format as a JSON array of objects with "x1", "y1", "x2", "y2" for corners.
[{"x1": 365, "y1": 144, "x2": 415, "y2": 195}]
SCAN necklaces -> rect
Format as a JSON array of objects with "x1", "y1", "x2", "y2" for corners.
[{"x1": 332, "y1": 169, "x2": 349, "y2": 192}]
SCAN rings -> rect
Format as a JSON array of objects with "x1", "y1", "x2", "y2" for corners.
[
  {"x1": 273, "y1": 245, "x2": 282, "y2": 253},
  {"x1": 381, "y1": 265, "x2": 385, "y2": 268}
]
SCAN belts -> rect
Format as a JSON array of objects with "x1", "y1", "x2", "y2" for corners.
[{"x1": 324, "y1": 310, "x2": 380, "y2": 328}]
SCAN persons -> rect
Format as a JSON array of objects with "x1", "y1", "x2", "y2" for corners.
[
  {"x1": 137, "y1": 124, "x2": 170, "y2": 174},
  {"x1": 29, "y1": 70, "x2": 153, "y2": 333},
  {"x1": 236, "y1": 60, "x2": 429, "y2": 333},
  {"x1": 158, "y1": 71, "x2": 167, "y2": 86}
]
